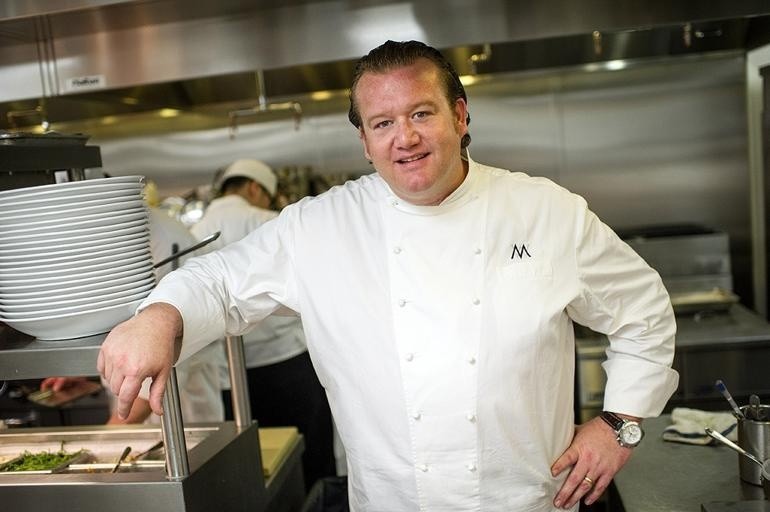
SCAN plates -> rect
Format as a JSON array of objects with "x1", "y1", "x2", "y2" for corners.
[{"x1": 0, "y1": 172, "x2": 157, "y2": 345}]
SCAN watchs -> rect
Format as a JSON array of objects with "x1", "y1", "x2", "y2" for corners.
[{"x1": 599, "y1": 411, "x2": 645, "y2": 448}]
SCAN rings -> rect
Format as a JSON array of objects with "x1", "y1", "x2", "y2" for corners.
[{"x1": 584, "y1": 476, "x2": 593, "y2": 484}]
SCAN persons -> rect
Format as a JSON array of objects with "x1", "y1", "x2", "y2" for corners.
[
  {"x1": 95, "y1": 37, "x2": 685, "y2": 512},
  {"x1": 186, "y1": 157, "x2": 337, "y2": 500},
  {"x1": 39, "y1": 173, "x2": 227, "y2": 424}
]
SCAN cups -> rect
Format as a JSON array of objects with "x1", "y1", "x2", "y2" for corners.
[{"x1": 732, "y1": 403, "x2": 769, "y2": 486}]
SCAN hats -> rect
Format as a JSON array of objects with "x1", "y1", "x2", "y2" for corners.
[{"x1": 216, "y1": 157, "x2": 277, "y2": 201}]
